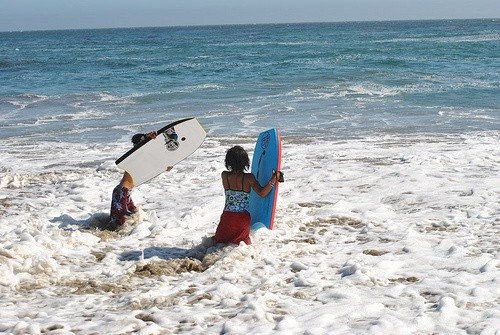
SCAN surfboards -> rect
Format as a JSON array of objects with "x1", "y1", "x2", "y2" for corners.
[
  {"x1": 115, "y1": 118, "x2": 207, "y2": 188},
  {"x1": 248, "y1": 128, "x2": 282, "y2": 230}
]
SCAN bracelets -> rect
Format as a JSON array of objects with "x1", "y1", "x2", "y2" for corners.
[{"x1": 267, "y1": 180, "x2": 274, "y2": 187}]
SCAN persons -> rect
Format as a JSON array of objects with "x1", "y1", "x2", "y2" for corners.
[
  {"x1": 110, "y1": 131, "x2": 158, "y2": 232},
  {"x1": 213, "y1": 146, "x2": 280, "y2": 246}
]
